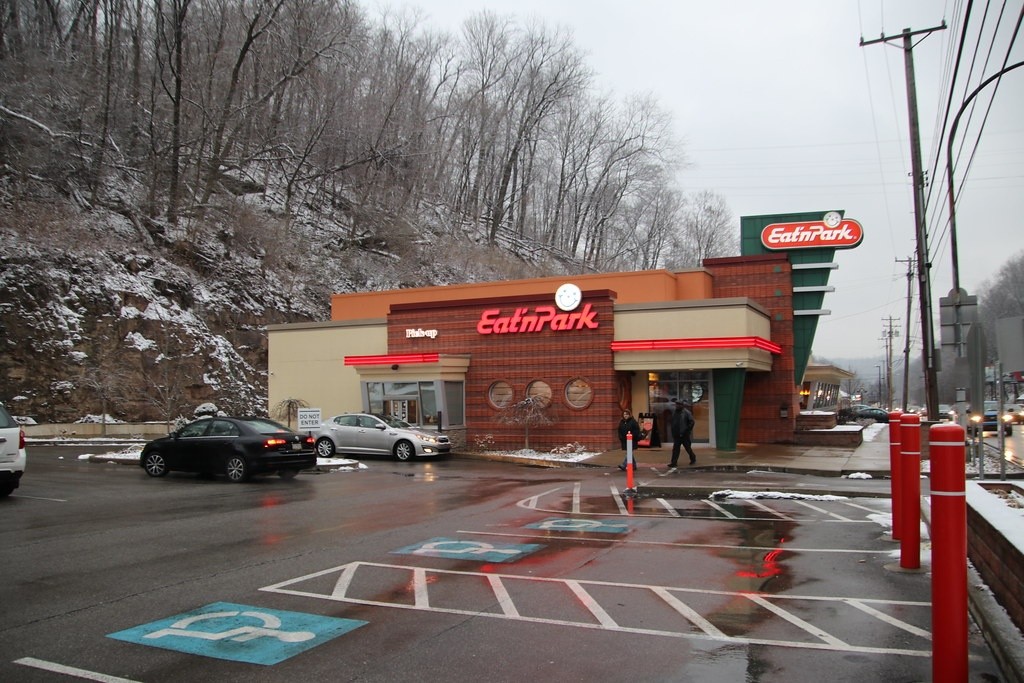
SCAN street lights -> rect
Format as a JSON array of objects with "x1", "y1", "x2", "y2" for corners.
[{"x1": 875, "y1": 364, "x2": 882, "y2": 407}]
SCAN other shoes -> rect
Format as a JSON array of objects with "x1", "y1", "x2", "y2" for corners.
[
  {"x1": 618, "y1": 464, "x2": 626, "y2": 471},
  {"x1": 689, "y1": 457, "x2": 697, "y2": 465},
  {"x1": 667, "y1": 463, "x2": 678, "y2": 467}
]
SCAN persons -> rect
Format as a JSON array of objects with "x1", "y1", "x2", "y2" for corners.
[
  {"x1": 618, "y1": 409, "x2": 640, "y2": 471},
  {"x1": 667, "y1": 401, "x2": 697, "y2": 467}
]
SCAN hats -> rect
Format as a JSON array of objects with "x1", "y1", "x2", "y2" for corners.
[{"x1": 675, "y1": 399, "x2": 684, "y2": 405}]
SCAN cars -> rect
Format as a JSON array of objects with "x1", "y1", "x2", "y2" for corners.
[
  {"x1": 138, "y1": 416, "x2": 318, "y2": 482},
  {"x1": 939, "y1": 405, "x2": 953, "y2": 420},
  {"x1": 836, "y1": 404, "x2": 890, "y2": 424},
  {"x1": 886, "y1": 405, "x2": 928, "y2": 417},
  {"x1": 0, "y1": 402, "x2": 26, "y2": 496},
  {"x1": 966, "y1": 400, "x2": 1013, "y2": 437},
  {"x1": 1004, "y1": 404, "x2": 1024, "y2": 424},
  {"x1": 316, "y1": 412, "x2": 452, "y2": 462}
]
